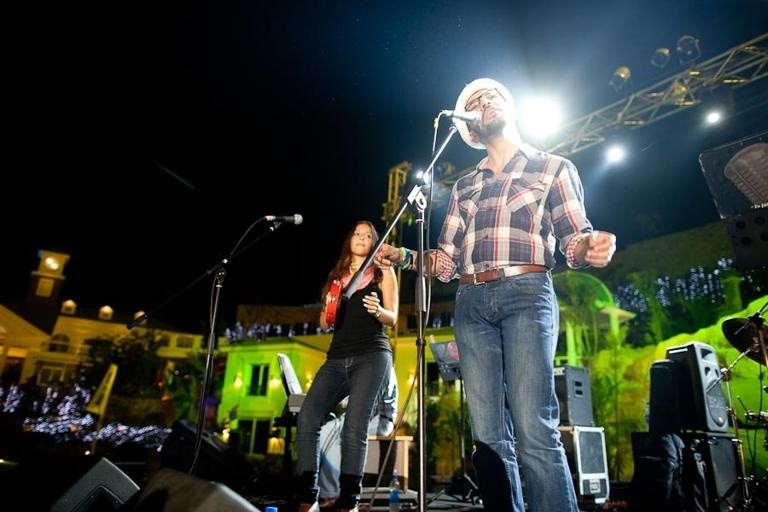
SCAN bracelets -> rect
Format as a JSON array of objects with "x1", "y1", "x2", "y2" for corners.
[
  {"x1": 373, "y1": 307, "x2": 383, "y2": 318},
  {"x1": 565, "y1": 233, "x2": 593, "y2": 270},
  {"x1": 394, "y1": 246, "x2": 413, "y2": 271}
]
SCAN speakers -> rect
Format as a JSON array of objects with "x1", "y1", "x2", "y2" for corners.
[
  {"x1": 553, "y1": 364, "x2": 595, "y2": 426},
  {"x1": 649, "y1": 340, "x2": 738, "y2": 430},
  {"x1": 160, "y1": 418, "x2": 255, "y2": 483},
  {"x1": 559, "y1": 425, "x2": 611, "y2": 505}
]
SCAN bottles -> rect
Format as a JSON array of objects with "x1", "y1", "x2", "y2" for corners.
[{"x1": 389, "y1": 475, "x2": 401, "y2": 512}]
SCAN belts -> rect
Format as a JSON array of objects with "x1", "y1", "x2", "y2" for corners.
[{"x1": 459, "y1": 264, "x2": 549, "y2": 286}]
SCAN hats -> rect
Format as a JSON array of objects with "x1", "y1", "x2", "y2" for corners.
[{"x1": 452, "y1": 76, "x2": 526, "y2": 151}]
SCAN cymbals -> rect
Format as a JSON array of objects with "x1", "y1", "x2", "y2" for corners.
[{"x1": 722, "y1": 318, "x2": 768, "y2": 365}]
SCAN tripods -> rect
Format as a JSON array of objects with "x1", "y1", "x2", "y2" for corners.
[{"x1": 426, "y1": 380, "x2": 481, "y2": 506}]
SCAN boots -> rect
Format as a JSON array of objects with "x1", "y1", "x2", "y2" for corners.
[
  {"x1": 286, "y1": 470, "x2": 323, "y2": 512},
  {"x1": 331, "y1": 472, "x2": 364, "y2": 512}
]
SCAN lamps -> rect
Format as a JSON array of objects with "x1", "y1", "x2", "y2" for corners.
[{"x1": 606, "y1": 36, "x2": 698, "y2": 98}]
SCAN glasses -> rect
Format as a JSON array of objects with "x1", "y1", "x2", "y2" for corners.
[{"x1": 464, "y1": 89, "x2": 499, "y2": 112}]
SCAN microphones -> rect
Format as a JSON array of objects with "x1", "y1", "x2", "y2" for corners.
[
  {"x1": 263, "y1": 212, "x2": 305, "y2": 225},
  {"x1": 442, "y1": 108, "x2": 482, "y2": 124}
]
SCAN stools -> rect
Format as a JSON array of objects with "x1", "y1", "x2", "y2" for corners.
[{"x1": 359, "y1": 434, "x2": 419, "y2": 511}]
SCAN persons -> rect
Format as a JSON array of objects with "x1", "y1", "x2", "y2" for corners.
[
  {"x1": 318, "y1": 364, "x2": 398, "y2": 509},
  {"x1": 273, "y1": 215, "x2": 399, "y2": 510},
  {"x1": 373, "y1": 76, "x2": 617, "y2": 509}
]
